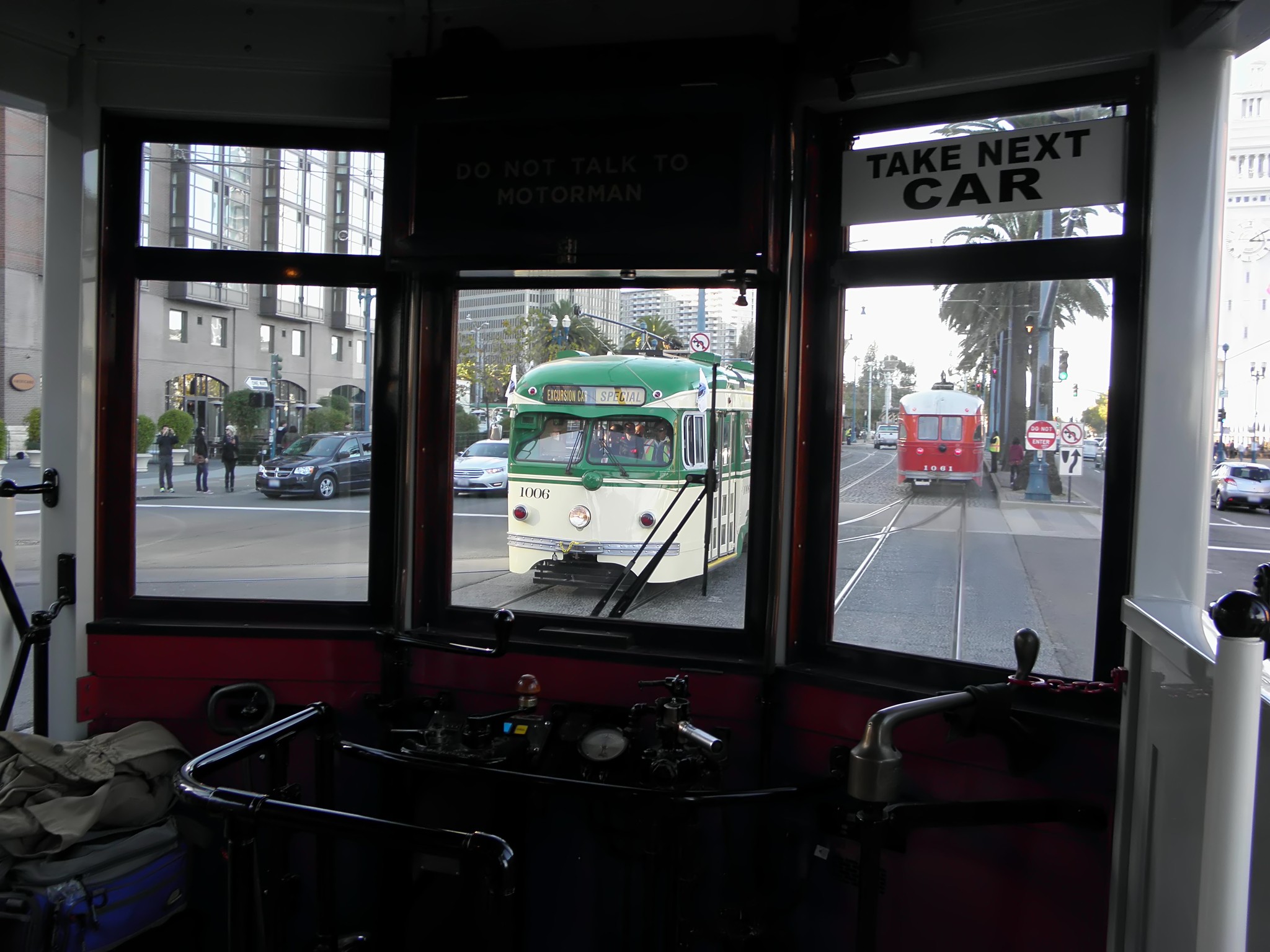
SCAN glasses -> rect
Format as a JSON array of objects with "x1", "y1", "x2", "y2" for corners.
[
  {"x1": 225, "y1": 429, "x2": 229, "y2": 431},
  {"x1": 653, "y1": 428, "x2": 666, "y2": 432}
]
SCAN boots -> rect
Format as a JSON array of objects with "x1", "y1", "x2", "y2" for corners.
[
  {"x1": 225, "y1": 479, "x2": 230, "y2": 492},
  {"x1": 230, "y1": 479, "x2": 234, "y2": 492}
]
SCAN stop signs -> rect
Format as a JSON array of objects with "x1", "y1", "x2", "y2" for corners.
[{"x1": 1026, "y1": 421, "x2": 1057, "y2": 451}]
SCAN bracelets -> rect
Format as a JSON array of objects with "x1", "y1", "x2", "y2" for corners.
[{"x1": 173, "y1": 433, "x2": 175, "y2": 434}]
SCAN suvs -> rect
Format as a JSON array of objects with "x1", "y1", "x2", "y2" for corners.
[{"x1": 255, "y1": 430, "x2": 371, "y2": 500}]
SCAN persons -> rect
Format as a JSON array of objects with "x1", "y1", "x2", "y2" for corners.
[
  {"x1": 591, "y1": 421, "x2": 673, "y2": 464},
  {"x1": 275, "y1": 420, "x2": 351, "y2": 455},
  {"x1": 1213, "y1": 440, "x2": 1245, "y2": 461},
  {"x1": 1008, "y1": 437, "x2": 1024, "y2": 485},
  {"x1": 843, "y1": 426, "x2": 853, "y2": 445},
  {"x1": 156, "y1": 425, "x2": 179, "y2": 492},
  {"x1": 214, "y1": 426, "x2": 240, "y2": 493},
  {"x1": 989, "y1": 430, "x2": 1000, "y2": 473},
  {"x1": 194, "y1": 426, "x2": 213, "y2": 494}
]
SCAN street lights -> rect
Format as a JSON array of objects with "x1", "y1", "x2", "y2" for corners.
[
  {"x1": 851, "y1": 354, "x2": 860, "y2": 443},
  {"x1": 866, "y1": 359, "x2": 874, "y2": 443},
  {"x1": 1215, "y1": 343, "x2": 1229, "y2": 464},
  {"x1": 548, "y1": 314, "x2": 571, "y2": 352},
  {"x1": 465, "y1": 318, "x2": 490, "y2": 409},
  {"x1": 1250, "y1": 362, "x2": 1267, "y2": 463}
]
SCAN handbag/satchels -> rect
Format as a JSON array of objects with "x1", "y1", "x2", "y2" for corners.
[{"x1": 193, "y1": 453, "x2": 205, "y2": 464}]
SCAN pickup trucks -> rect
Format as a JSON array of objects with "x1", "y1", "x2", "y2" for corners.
[{"x1": 873, "y1": 423, "x2": 899, "y2": 450}]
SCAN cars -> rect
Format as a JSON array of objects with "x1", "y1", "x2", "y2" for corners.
[
  {"x1": 1082, "y1": 436, "x2": 1107, "y2": 469},
  {"x1": 1052, "y1": 437, "x2": 1061, "y2": 454},
  {"x1": 452, "y1": 438, "x2": 508, "y2": 495},
  {"x1": 469, "y1": 408, "x2": 495, "y2": 432},
  {"x1": 1210, "y1": 461, "x2": 1270, "y2": 511}
]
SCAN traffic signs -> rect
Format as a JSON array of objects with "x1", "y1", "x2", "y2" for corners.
[{"x1": 244, "y1": 376, "x2": 271, "y2": 391}]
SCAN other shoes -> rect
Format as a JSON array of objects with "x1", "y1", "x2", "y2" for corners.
[
  {"x1": 1010, "y1": 484, "x2": 1014, "y2": 488},
  {"x1": 989, "y1": 471, "x2": 997, "y2": 473},
  {"x1": 197, "y1": 489, "x2": 203, "y2": 493},
  {"x1": 167, "y1": 488, "x2": 174, "y2": 493},
  {"x1": 159, "y1": 487, "x2": 164, "y2": 492},
  {"x1": 203, "y1": 490, "x2": 214, "y2": 494}
]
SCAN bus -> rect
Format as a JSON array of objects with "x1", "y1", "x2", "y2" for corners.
[
  {"x1": 491, "y1": 310, "x2": 757, "y2": 591},
  {"x1": 897, "y1": 370, "x2": 989, "y2": 493}
]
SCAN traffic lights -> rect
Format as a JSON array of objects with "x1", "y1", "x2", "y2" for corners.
[
  {"x1": 272, "y1": 353, "x2": 282, "y2": 381},
  {"x1": 1218, "y1": 409, "x2": 1227, "y2": 423},
  {"x1": 1072, "y1": 383, "x2": 1078, "y2": 396},
  {"x1": 1059, "y1": 349, "x2": 1069, "y2": 380},
  {"x1": 1023, "y1": 314, "x2": 1036, "y2": 335}
]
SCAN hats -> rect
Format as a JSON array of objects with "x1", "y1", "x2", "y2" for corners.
[
  {"x1": 226, "y1": 425, "x2": 234, "y2": 432},
  {"x1": 196, "y1": 427, "x2": 205, "y2": 432}
]
